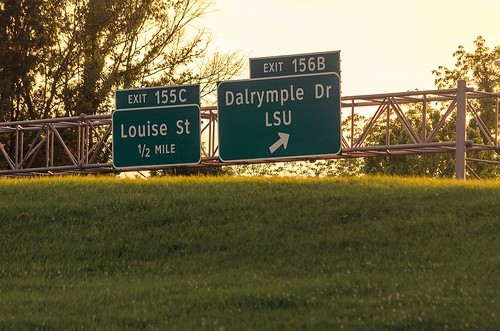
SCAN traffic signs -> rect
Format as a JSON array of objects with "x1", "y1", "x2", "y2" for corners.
[
  {"x1": 112, "y1": 84, "x2": 201, "y2": 166},
  {"x1": 217, "y1": 50, "x2": 342, "y2": 163}
]
